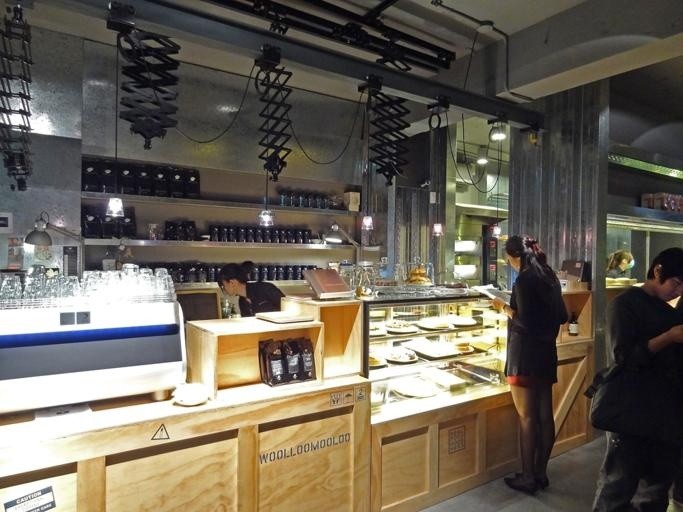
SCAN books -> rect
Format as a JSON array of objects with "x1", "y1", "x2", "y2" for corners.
[
  {"x1": 302, "y1": 268, "x2": 352, "y2": 298},
  {"x1": 470, "y1": 282, "x2": 511, "y2": 305}
]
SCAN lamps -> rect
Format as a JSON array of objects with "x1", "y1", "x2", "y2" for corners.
[
  {"x1": 420, "y1": 94, "x2": 454, "y2": 238},
  {"x1": 352, "y1": 72, "x2": 382, "y2": 232},
  {"x1": 490, "y1": 123, "x2": 508, "y2": 141},
  {"x1": 92, "y1": 1, "x2": 137, "y2": 217},
  {"x1": 22, "y1": 209, "x2": 83, "y2": 279},
  {"x1": 253, "y1": 43, "x2": 283, "y2": 228},
  {"x1": 474, "y1": 145, "x2": 489, "y2": 166},
  {"x1": 323, "y1": 223, "x2": 362, "y2": 285},
  {"x1": 485, "y1": 114, "x2": 502, "y2": 238}
]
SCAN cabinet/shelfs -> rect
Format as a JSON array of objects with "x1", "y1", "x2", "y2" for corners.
[
  {"x1": 78, "y1": 187, "x2": 360, "y2": 293},
  {"x1": 551, "y1": 288, "x2": 593, "y2": 461},
  {"x1": 350, "y1": 283, "x2": 531, "y2": 512},
  {"x1": 276, "y1": 290, "x2": 366, "y2": 381},
  {"x1": 182, "y1": 291, "x2": 328, "y2": 401}
]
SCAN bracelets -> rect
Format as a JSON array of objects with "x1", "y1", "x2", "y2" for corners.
[{"x1": 501, "y1": 304, "x2": 507, "y2": 313}]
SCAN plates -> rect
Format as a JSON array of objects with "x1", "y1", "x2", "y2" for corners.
[
  {"x1": 370, "y1": 315, "x2": 476, "y2": 337},
  {"x1": 369, "y1": 340, "x2": 475, "y2": 369},
  {"x1": 435, "y1": 287, "x2": 467, "y2": 295},
  {"x1": 372, "y1": 284, "x2": 433, "y2": 295},
  {"x1": 391, "y1": 378, "x2": 437, "y2": 398}
]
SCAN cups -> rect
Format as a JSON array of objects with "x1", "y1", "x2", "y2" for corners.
[
  {"x1": 559, "y1": 280, "x2": 567, "y2": 291},
  {"x1": 0, "y1": 262, "x2": 176, "y2": 309}
]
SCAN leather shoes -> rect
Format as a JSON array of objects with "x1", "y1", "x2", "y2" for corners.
[
  {"x1": 512, "y1": 469, "x2": 549, "y2": 489},
  {"x1": 503, "y1": 476, "x2": 540, "y2": 494}
]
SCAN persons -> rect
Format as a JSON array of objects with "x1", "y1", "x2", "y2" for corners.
[
  {"x1": 592, "y1": 247, "x2": 683, "y2": 511},
  {"x1": 492, "y1": 234, "x2": 568, "y2": 496},
  {"x1": 217, "y1": 261, "x2": 286, "y2": 317}
]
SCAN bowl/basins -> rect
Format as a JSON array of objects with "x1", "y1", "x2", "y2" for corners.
[{"x1": 311, "y1": 239, "x2": 321, "y2": 244}]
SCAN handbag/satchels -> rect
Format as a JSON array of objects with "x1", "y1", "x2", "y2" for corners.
[{"x1": 584, "y1": 358, "x2": 683, "y2": 439}]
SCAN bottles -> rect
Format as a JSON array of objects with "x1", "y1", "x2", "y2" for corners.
[
  {"x1": 568, "y1": 312, "x2": 578, "y2": 335},
  {"x1": 208, "y1": 224, "x2": 311, "y2": 243},
  {"x1": 146, "y1": 223, "x2": 158, "y2": 240},
  {"x1": 170, "y1": 261, "x2": 323, "y2": 283},
  {"x1": 338, "y1": 262, "x2": 375, "y2": 300},
  {"x1": 279, "y1": 191, "x2": 330, "y2": 209}
]
